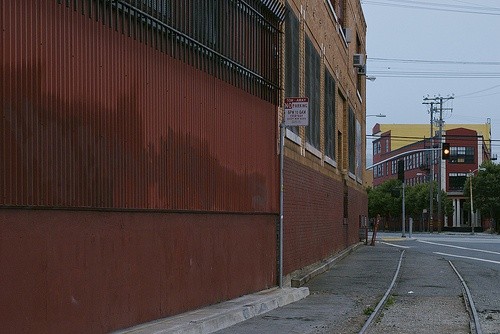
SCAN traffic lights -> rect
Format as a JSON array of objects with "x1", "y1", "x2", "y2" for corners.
[{"x1": 442, "y1": 142, "x2": 450, "y2": 160}]
